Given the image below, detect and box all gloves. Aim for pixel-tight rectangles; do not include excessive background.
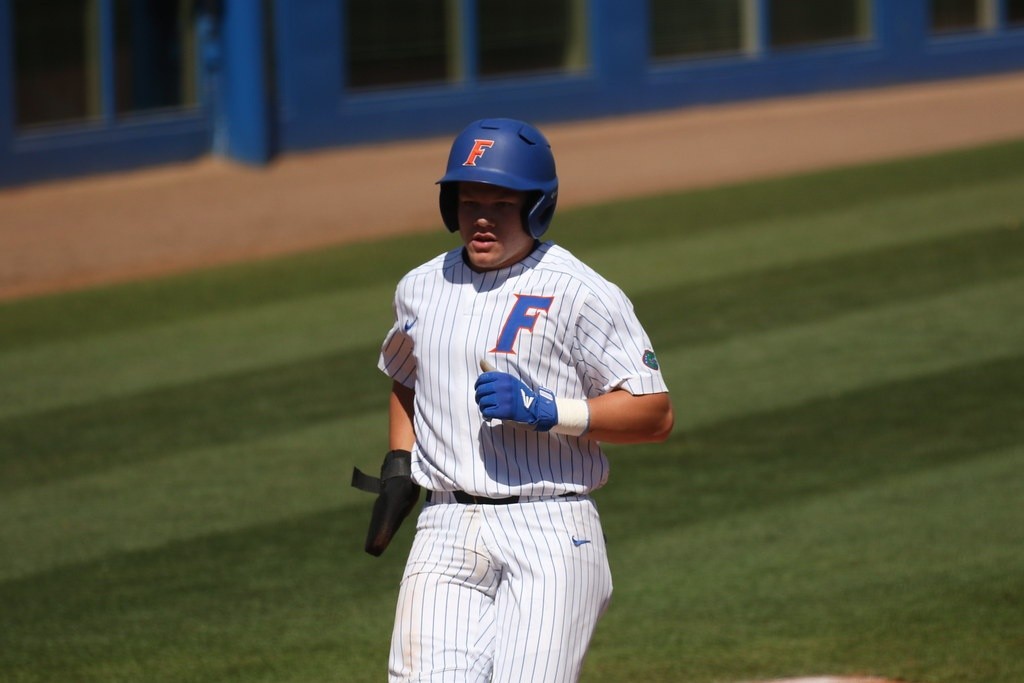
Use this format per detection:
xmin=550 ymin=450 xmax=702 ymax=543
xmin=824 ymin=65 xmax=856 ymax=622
xmin=473 ymin=359 xmax=559 ymax=432
xmin=350 ymin=448 xmax=422 ymax=557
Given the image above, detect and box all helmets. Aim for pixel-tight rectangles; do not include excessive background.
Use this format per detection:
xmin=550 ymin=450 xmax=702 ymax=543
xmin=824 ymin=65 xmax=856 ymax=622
xmin=435 ymin=119 xmax=559 ymax=240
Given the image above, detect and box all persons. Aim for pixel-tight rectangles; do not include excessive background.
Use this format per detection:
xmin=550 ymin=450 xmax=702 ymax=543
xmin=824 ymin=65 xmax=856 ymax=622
xmin=365 ymin=117 xmax=674 ymax=683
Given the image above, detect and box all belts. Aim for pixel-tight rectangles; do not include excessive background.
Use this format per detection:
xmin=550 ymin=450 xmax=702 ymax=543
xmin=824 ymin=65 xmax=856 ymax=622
xmin=426 ymin=490 xmax=578 ymax=506
